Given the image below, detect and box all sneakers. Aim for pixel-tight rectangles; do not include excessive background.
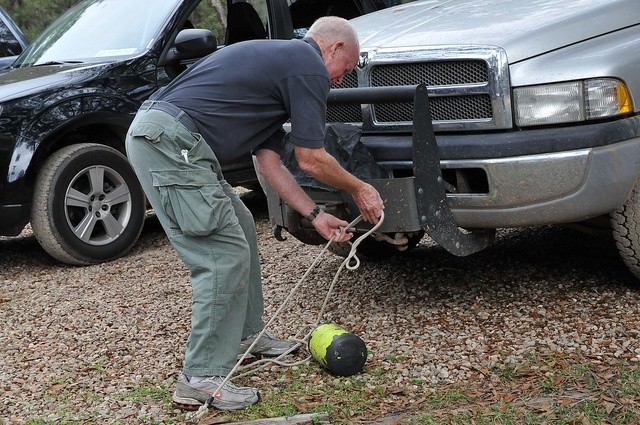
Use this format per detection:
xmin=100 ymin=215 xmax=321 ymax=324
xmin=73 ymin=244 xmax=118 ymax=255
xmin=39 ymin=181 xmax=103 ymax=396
xmin=172 ymin=374 xmax=261 ymax=411
xmin=236 ymin=330 xmax=302 ymax=364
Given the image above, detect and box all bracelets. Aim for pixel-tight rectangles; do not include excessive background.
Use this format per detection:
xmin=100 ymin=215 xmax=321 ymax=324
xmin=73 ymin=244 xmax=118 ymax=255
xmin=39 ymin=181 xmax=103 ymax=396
xmin=305 ymin=206 xmax=320 ymax=222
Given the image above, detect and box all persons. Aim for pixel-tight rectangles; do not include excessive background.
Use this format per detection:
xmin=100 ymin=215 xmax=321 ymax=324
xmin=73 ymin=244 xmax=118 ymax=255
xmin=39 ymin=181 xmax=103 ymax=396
xmin=124 ymin=16 xmax=385 ymax=412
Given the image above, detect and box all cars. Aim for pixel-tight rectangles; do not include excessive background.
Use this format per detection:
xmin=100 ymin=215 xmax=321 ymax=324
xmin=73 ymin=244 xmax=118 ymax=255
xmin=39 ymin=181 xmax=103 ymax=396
xmin=0 ymin=0 xmax=412 ymax=265
xmin=252 ymin=0 xmax=639 ymax=282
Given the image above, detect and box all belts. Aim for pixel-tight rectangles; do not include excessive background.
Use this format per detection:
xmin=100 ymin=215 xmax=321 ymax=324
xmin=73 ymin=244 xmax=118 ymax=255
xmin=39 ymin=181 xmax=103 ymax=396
xmin=139 ymin=101 xmax=199 ymax=133
